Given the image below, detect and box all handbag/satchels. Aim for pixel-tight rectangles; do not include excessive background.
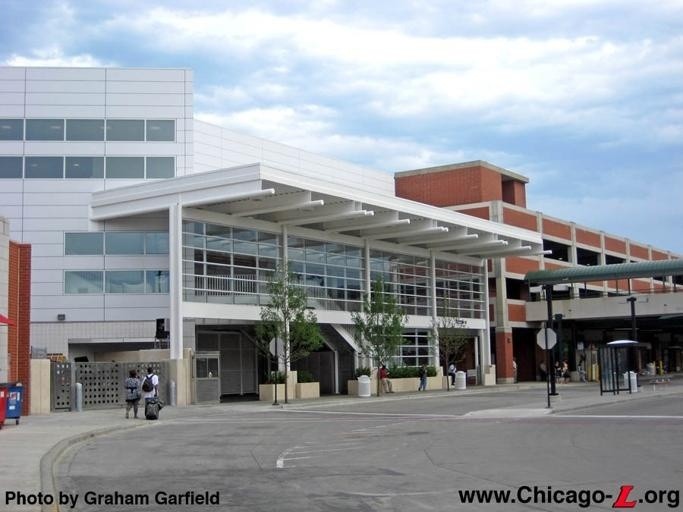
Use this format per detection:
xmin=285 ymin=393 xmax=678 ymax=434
xmin=145 ymin=397 xmax=160 ymax=404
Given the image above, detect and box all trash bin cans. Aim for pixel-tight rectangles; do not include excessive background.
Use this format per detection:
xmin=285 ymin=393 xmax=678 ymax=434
xmin=455 ymin=371 xmax=467 ymax=390
xmin=622 ymin=371 xmax=638 ymax=393
xmin=0 ymin=383 xmax=24 ymax=430
xmin=357 ymin=375 xmax=371 ymax=398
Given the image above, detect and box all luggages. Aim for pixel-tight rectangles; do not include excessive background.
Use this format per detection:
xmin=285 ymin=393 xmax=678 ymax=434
xmin=145 ymin=404 xmax=159 ymax=420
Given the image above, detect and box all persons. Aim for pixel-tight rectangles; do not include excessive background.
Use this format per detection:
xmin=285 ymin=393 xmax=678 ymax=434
xmin=539 ymin=358 xmax=588 ymax=383
xmin=124 ymin=368 xmax=141 ymax=419
xmin=512 ymin=357 xmax=517 ymax=384
xmin=448 ymin=362 xmax=457 ymax=385
xmin=417 ymin=363 xmax=427 ymax=391
xmin=379 ymin=363 xmax=395 ymax=393
xmin=139 ymin=366 xmax=160 ymax=418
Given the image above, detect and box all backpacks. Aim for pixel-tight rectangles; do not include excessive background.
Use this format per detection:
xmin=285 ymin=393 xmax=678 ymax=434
xmin=143 ymin=375 xmax=154 ymax=392
